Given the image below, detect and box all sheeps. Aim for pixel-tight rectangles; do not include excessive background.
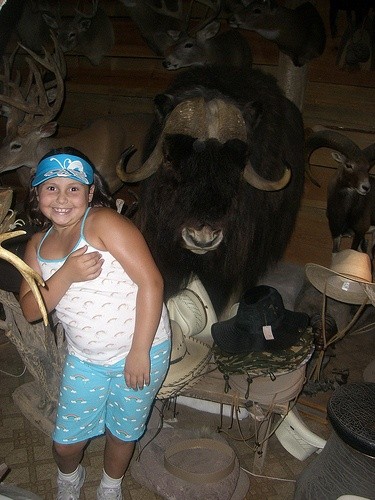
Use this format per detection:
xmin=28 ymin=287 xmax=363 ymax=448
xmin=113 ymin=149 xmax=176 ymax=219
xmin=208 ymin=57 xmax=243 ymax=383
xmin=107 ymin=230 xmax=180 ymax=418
xmin=306 ymin=130 xmax=375 ymax=259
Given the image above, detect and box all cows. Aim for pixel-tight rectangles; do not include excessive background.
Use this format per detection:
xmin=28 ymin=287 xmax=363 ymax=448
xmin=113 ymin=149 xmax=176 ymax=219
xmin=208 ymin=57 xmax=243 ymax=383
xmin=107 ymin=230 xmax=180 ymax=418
xmin=115 ymin=63 xmax=306 ymax=320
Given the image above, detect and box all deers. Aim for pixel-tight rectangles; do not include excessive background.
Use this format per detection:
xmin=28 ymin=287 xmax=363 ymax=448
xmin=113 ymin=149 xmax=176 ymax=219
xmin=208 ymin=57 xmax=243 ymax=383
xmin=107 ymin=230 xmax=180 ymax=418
xmin=145 ymin=1 xmax=253 ymax=70
xmin=226 ymin=0 xmax=325 ymax=67
xmin=30 ymin=0 xmax=114 ymax=79
xmin=0 ymin=42 xmax=155 ymax=196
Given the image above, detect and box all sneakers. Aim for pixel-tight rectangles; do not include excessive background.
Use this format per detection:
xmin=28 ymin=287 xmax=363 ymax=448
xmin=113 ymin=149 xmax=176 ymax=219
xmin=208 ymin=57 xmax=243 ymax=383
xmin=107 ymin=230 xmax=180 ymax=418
xmin=95 ymin=476 xmax=126 ymax=500
xmin=54 ymin=463 xmax=87 ymax=499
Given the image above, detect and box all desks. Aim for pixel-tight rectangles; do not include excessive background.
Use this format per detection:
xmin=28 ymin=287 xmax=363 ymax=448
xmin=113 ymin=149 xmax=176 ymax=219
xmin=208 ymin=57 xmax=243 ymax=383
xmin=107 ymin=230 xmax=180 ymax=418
xmin=185 ymin=365 xmax=306 ymax=476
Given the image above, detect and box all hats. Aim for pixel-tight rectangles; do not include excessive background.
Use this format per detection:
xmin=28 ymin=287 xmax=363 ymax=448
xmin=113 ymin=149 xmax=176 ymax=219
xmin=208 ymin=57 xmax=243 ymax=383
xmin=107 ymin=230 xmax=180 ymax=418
xmin=211 ymin=285 xmax=317 ymax=403
xmin=273 ymin=403 xmax=326 ymax=461
xmin=305 ymin=248 xmax=375 ymax=305
xmin=32 ymin=154 xmax=93 ymax=187
xmin=151 ymin=266 xmax=218 ymax=399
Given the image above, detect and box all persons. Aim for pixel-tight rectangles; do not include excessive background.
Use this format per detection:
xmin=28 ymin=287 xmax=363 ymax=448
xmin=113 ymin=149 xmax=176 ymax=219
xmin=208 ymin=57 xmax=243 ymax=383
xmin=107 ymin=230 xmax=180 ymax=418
xmin=20 ymin=146 xmax=172 ymax=500
